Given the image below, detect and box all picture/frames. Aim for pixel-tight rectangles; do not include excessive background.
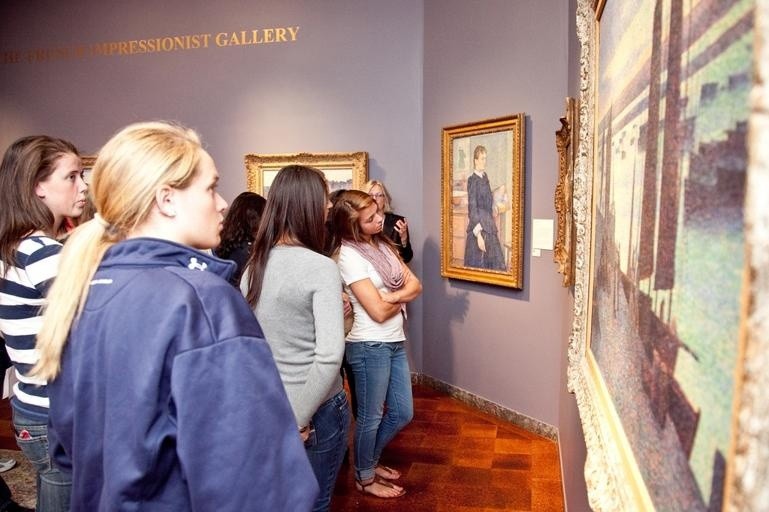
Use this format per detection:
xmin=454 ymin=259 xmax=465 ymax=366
xmin=244 ymin=150 xmax=369 ymax=199
xmin=440 ymin=112 xmax=528 ymax=290
xmin=553 ymin=0 xmax=769 ymax=512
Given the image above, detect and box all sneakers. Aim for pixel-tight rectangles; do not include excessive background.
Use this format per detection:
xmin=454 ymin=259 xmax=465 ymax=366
xmin=0 ymin=458 xmax=17 ymax=473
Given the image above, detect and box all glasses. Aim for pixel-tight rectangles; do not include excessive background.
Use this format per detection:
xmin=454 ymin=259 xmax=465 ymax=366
xmin=369 ymin=194 xmax=383 ymax=198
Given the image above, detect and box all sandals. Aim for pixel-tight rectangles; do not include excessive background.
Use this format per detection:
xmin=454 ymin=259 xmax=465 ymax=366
xmin=356 ymin=463 xmax=406 ymax=498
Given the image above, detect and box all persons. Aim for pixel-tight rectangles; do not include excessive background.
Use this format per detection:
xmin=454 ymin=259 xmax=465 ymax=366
xmin=0 ymin=134 xmax=90 ymax=512
xmin=216 ymin=164 xmax=426 ymax=511
xmin=46 ymin=120 xmax=322 ymax=512
xmin=462 ymin=146 xmax=508 ymax=274
